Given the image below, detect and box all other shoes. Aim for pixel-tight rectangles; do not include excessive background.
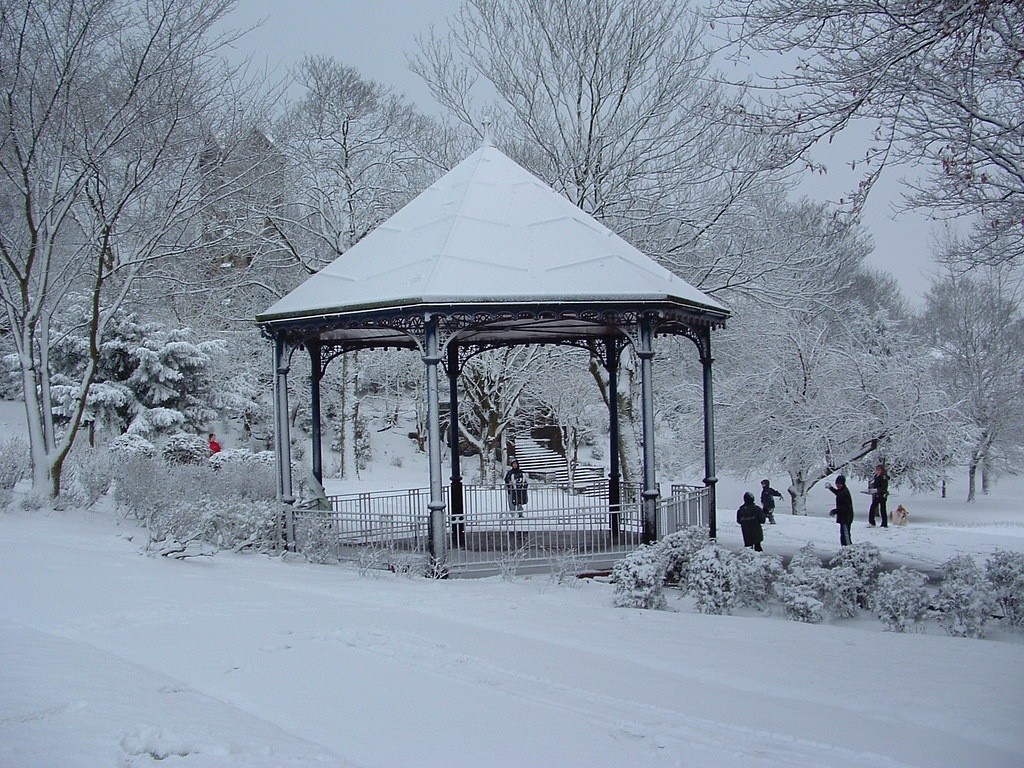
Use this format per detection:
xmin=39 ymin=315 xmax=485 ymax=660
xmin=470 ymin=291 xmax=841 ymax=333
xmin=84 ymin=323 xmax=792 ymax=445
xmin=881 ymin=526 xmax=886 ymax=528
xmin=866 ymin=524 xmax=876 ymax=528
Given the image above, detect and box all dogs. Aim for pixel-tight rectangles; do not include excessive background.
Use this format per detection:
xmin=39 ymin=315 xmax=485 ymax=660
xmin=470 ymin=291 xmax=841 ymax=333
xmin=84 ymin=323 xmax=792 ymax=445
xmin=890 ymin=504 xmax=909 ymax=524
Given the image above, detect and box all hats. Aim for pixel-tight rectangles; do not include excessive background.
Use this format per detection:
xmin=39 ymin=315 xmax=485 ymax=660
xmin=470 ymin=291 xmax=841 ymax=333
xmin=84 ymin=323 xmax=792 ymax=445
xmin=836 ymin=475 xmax=846 ymax=483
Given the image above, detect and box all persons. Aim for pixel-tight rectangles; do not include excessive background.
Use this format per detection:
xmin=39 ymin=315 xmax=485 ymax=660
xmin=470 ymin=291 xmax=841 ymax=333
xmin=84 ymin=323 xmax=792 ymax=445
xmin=209 ymin=433 xmax=222 ymax=457
xmin=760 ymin=479 xmax=783 ymax=524
xmin=505 ymin=458 xmax=528 ymax=518
xmin=860 ymin=465 xmax=888 ymax=529
xmin=824 ymin=475 xmax=854 ymax=547
xmin=736 ymin=492 xmax=766 ymax=553
